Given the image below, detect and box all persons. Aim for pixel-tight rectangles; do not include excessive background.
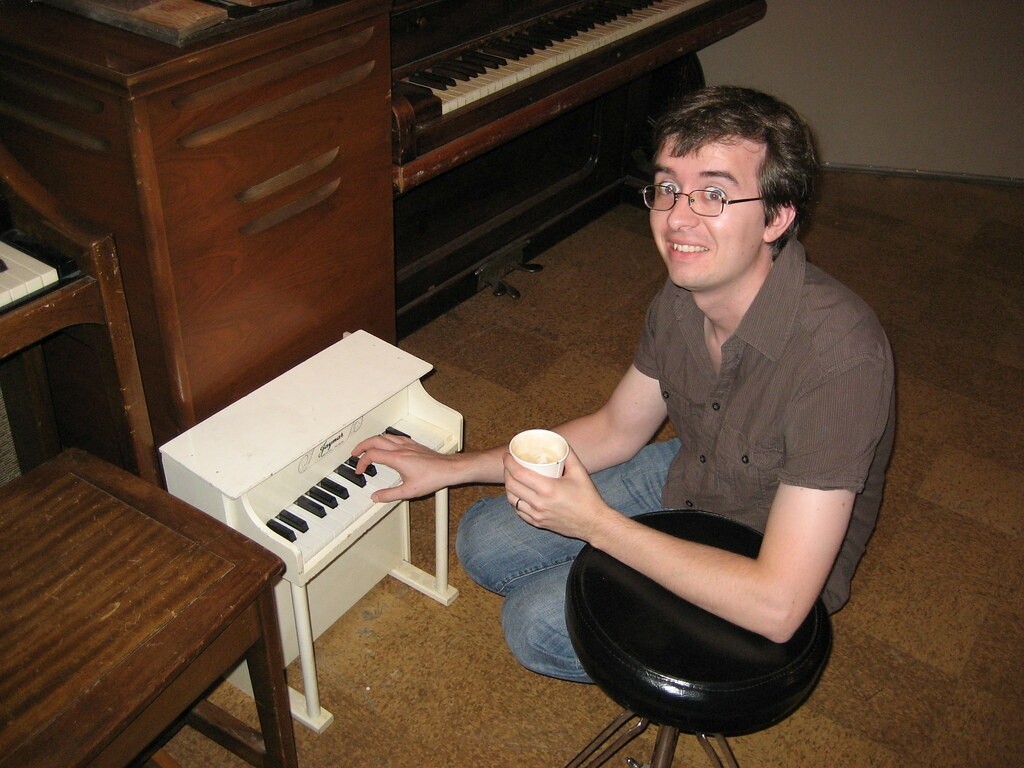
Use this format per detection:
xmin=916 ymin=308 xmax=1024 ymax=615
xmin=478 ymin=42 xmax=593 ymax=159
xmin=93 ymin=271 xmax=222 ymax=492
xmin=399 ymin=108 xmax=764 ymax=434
xmin=352 ymin=86 xmax=897 ymax=682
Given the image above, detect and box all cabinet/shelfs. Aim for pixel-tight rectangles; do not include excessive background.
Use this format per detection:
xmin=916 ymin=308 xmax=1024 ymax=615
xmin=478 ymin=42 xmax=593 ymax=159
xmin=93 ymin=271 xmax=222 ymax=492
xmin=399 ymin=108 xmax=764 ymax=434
xmin=0 ymin=0 xmax=397 ymax=493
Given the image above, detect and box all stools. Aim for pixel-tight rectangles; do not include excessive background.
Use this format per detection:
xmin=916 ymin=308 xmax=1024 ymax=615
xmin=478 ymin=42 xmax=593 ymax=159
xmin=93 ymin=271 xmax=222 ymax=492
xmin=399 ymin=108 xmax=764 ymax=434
xmin=563 ymin=508 xmax=833 ymax=768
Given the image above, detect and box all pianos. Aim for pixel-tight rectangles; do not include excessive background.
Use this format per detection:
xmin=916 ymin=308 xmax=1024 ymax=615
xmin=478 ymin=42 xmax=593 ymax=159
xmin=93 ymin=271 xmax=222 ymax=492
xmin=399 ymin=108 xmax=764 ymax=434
xmin=157 ymin=328 xmax=466 ymax=737
xmin=0 ymin=142 xmax=163 ymax=489
xmin=392 ymin=0 xmax=770 ymax=343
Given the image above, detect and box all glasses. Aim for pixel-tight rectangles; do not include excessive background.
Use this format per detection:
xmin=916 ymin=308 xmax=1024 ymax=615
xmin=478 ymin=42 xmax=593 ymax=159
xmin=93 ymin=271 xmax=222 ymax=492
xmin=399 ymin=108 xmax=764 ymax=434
xmin=641 ymin=185 xmax=767 ymax=217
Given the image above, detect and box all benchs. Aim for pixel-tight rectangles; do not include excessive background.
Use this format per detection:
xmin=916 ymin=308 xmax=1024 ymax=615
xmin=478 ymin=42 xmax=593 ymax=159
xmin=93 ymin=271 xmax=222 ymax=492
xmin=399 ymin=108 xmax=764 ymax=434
xmin=0 ymin=445 xmax=299 ymax=768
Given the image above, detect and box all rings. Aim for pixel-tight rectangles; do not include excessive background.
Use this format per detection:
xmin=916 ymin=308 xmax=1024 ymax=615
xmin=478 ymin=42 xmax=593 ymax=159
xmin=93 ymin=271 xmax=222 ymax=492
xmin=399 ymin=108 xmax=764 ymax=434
xmin=516 ymin=498 xmax=521 ymax=510
